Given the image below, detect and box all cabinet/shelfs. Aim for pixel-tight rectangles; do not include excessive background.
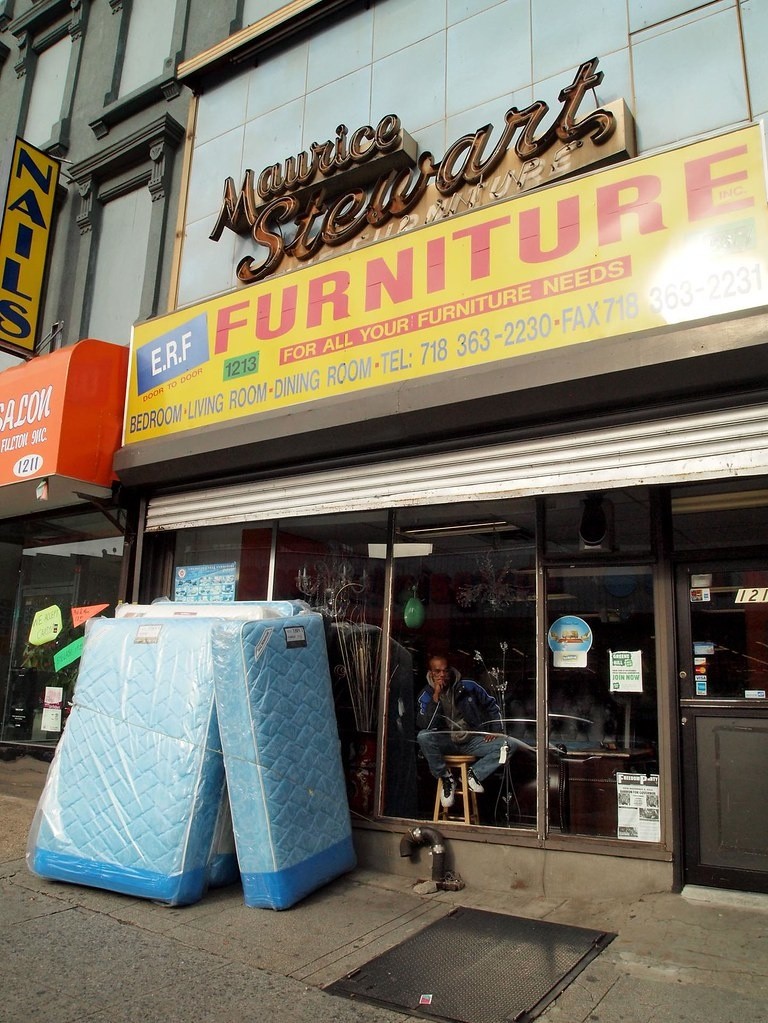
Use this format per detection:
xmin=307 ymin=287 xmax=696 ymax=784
xmin=560 ymin=745 xmax=653 ymax=837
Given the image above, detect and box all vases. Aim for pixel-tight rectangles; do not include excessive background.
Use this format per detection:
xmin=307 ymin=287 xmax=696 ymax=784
xmin=345 ymin=729 xmax=378 ymax=817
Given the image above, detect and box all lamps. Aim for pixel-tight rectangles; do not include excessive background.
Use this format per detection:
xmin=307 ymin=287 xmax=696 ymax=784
xmin=404 ymin=584 xmax=426 ymax=628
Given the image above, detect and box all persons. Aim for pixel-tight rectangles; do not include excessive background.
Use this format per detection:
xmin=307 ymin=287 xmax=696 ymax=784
xmin=619 ymin=794 xmax=658 ymax=836
xmin=415 ymin=654 xmax=517 ymax=807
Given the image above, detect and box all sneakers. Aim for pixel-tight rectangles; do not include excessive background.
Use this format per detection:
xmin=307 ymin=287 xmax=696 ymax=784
xmin=459 ymin=769 xmax=485 ymax=793
xmin=440 ymin=777 xmax=457 ymax=807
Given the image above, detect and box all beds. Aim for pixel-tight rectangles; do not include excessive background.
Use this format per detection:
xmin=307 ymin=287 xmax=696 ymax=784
xmin=27 ymin=600 xmax=358 ymax=913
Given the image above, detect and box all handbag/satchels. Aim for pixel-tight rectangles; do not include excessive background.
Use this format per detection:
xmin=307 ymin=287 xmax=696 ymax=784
xmin=450 ymin=720 xmax=470 ymax=744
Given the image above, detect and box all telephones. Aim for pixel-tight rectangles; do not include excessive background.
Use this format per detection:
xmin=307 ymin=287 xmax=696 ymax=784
xmin=555 ymin=743 xmax=568 ymax=754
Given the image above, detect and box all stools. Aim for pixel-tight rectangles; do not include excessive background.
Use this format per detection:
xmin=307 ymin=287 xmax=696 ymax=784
xmin=433 ymin=754 xmax=480 ymax=825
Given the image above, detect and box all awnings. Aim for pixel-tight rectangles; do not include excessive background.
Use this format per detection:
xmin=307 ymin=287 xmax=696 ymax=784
xmin=0 ymin=339 xmax=130 ymax=519
